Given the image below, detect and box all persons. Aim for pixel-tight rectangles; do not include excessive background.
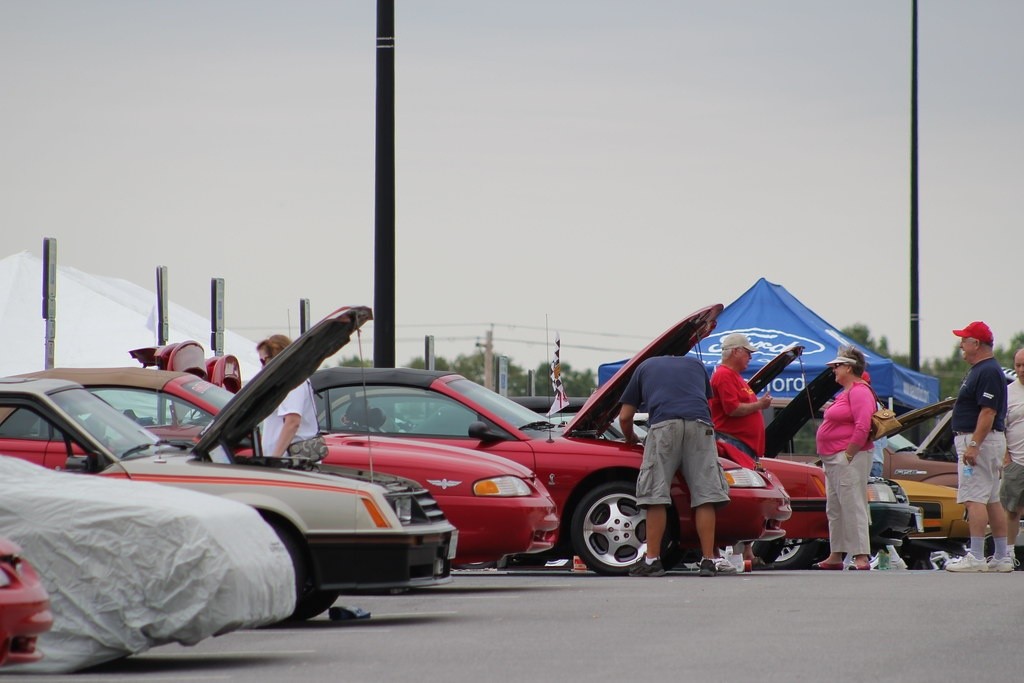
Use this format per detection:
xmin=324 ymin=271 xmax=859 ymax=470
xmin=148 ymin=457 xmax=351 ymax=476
xmin=861 ymin=373 xmax=886 ymax=478
xmin=619 ymin=354 xmax=730 ymax=576
xmin=812 ymin=347 xmax=877 ymax=571
xmin=946 ymin=321 xmax=1013 ymax=573
xmin=257 ymin=335 xmax=321 ymax=463
xmin=707 ymin=334 xmax=772 ymax=570
xmin=1000 ymin=348 xmax=1024 ymax=567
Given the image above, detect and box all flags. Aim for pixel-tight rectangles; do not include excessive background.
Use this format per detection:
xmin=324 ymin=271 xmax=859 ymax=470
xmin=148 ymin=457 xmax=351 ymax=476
xmin=546 ymin=333 xmax=569 ymax=416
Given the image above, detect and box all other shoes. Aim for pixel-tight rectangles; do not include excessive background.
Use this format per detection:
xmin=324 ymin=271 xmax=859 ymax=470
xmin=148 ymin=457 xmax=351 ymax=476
xmin=869 ymin=553 xmax=908 ymax=570
xmin=812 ymin=562 xmax=844 ymax=570
xmin=752 ymin=556 xmax=776 ymax=569
xmin=848 ymin=564 xmax=871 ymax=570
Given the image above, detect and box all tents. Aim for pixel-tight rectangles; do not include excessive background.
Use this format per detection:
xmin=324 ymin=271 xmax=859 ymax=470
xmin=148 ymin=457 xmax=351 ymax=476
xmin=598 ymin=278 xmax=938 ymax=418
xmin=1 ymin=249 xmax=265 ymax=423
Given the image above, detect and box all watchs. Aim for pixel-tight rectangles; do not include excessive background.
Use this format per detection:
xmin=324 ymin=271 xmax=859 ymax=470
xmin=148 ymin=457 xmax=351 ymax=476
xmin=970 ymin=441 xmax=976 ymax=446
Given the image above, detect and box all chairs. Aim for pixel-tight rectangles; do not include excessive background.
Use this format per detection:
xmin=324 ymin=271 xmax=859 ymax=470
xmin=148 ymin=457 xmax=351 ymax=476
xmin=343 ymin=398 xmax=369 ymax=432
xmin=368 ymin=407 xmax=388 ymax=433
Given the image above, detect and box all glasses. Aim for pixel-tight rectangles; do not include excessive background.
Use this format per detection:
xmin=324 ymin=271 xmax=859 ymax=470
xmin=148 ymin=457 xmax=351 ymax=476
xmin=260 ymin=355 xmax=269 ymax=366
xmin=835 ymin=363 xmax=844 ymax=368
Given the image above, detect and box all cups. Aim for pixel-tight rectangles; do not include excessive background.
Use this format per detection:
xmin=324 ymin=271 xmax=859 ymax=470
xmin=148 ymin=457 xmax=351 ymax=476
xmin=573 ymin=555 xmax=588 ymax=571
xmin=744 ymin=560 xmax=753 ymax=572
xmin=725 ymin=553 xmax=743 ymax=572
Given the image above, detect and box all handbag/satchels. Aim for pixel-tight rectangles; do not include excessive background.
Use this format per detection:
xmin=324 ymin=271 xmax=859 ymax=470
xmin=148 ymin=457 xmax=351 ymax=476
xmin=288 ymin=435 xmax=329 ymax=466
xmin=848 ymin=382 xmax=902 ymax=441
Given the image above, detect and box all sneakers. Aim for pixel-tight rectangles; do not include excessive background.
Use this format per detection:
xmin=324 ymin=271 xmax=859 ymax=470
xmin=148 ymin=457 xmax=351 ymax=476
xmin=628 ymin=552 xmax=665 ymax=577
xmin=700 ymin=557 xmax=717 ymax=576
xmin=946 ymin=552 xmax=988 ymax=573
xmin=989 ymin=556 xmax=1020 ymax=572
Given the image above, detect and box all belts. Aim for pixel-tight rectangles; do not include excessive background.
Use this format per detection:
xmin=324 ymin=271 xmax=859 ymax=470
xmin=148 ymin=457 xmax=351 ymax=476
xmin=953 ymin=430 xmax=970 ymax=436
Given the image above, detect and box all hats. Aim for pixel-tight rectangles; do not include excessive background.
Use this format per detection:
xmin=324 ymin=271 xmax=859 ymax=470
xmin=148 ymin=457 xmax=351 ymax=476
xmin=952 ymin=320 xmax=994 ymax=343
xmin=722 ymin=334 xmax=759 ymax=353
xmin=826 ymin=355 xmax=866 ymax=367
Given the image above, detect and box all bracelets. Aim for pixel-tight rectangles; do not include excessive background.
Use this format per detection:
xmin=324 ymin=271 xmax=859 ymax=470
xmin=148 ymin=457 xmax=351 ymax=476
xmin=845 ymin=453 xmax=852 ymax=460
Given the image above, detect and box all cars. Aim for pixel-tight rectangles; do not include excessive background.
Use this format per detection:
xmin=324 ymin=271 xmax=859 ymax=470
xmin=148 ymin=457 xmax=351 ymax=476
xmin=142 ymin=365 xmax=794 ymax=578
xmin=0 ymin=454 xmax=298 ymax=676
xmin=319 ymin=397 xmax=1024 ymax=578
xmin=2 ymin=366 xmax=562 ymax=572
xmin=1 ymin=304 xmax=458 ymax=632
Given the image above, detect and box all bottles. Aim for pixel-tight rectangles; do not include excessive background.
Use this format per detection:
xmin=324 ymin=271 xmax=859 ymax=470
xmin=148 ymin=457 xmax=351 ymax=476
xmin=879 ymin=549 xmax=889 ymax=569
